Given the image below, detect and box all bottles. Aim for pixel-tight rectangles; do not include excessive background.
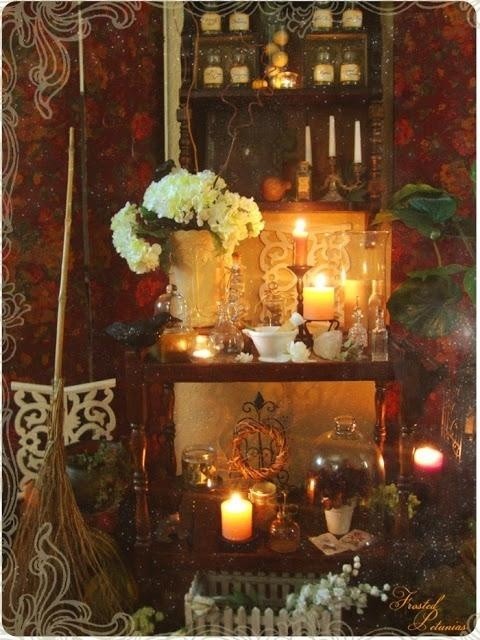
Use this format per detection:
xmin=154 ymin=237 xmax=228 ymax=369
xmin=312 ymin=1 xmax=364 ymax=87
xmin=371 ymin=308 xmax=388 ymax=362
xmin=201 ymin=1 xmax=250 ymax=88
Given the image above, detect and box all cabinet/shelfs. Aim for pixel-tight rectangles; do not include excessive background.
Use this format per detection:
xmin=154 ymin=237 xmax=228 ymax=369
xmin=116 ymin=1 xmax=450 ymax=632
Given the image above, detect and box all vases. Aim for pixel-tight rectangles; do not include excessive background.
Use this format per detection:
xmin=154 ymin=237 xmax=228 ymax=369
xmin=161 ymin=228 xmax=230 ymax=329
xmin=383 ymin=511 xmax=405 ymax=534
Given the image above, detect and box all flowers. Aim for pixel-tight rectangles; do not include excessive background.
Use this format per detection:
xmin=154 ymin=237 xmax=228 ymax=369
xmin=110 ymin=167 xmax=267 ymax=277
xmin=373 ymin=483 xmax=418 ymax=520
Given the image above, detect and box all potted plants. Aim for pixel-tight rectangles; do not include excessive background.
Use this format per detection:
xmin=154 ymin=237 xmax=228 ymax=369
xmin=344 ymin=278 xmax=369 ymax=337
xmin=312 ymin=465 xmax=372 ymax=534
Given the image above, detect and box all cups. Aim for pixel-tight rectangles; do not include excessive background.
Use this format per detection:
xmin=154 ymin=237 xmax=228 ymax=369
xmin=182 ymin=445 xmax=218 ymax=493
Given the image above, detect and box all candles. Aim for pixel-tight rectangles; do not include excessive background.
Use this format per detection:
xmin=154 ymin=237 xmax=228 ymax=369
xmin=304 ymin=124 xmax=313 ymax=168
xmin=302 ymin=273 xmax=335 ymax=322
xmin=221 ymin=490 xmax=253 ymax=542
xmin=293 ymin=217 xmax=309 ymax=268
xmin=328 ymin=115 xmax=337 ymax=157
xmin=354 ymin=120 xmax=363 ymax=164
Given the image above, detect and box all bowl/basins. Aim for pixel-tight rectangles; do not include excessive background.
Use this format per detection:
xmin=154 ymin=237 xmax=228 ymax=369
xmin=242 ymin=326 xmax=298 ymax=362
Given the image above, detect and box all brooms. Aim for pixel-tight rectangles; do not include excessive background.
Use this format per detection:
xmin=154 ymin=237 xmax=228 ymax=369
xmin=1 ymin=127 xmax=131 ymax=636
